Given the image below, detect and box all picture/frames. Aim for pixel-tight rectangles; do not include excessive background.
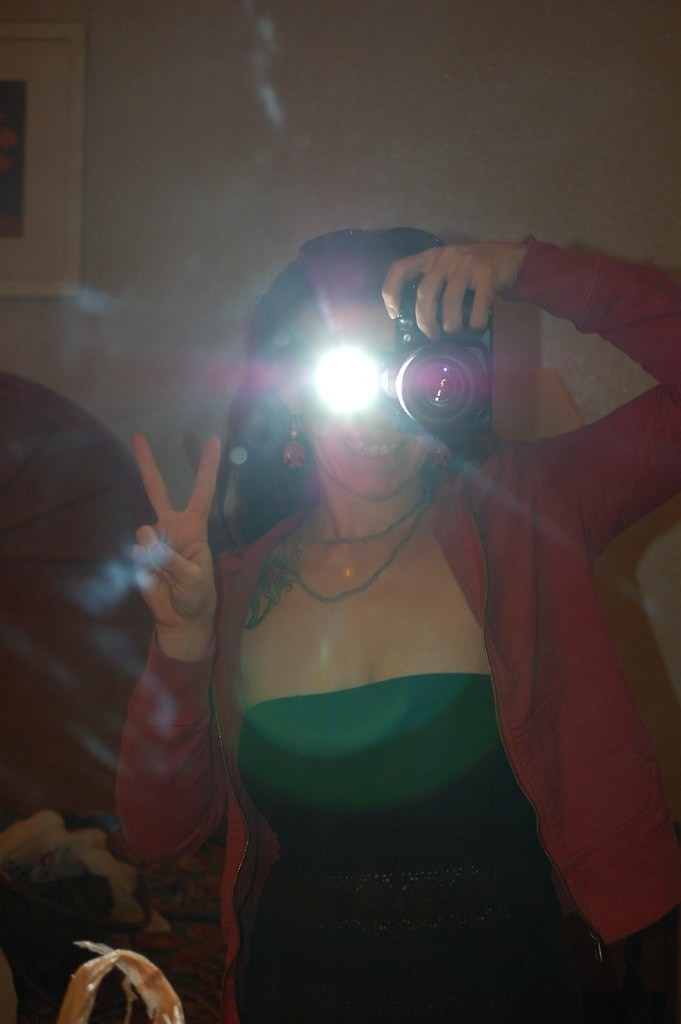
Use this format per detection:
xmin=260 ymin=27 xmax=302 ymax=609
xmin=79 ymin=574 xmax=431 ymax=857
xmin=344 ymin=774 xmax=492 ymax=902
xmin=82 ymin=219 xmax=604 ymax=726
xmin=0 ymin=21 xmax=89 ymax=300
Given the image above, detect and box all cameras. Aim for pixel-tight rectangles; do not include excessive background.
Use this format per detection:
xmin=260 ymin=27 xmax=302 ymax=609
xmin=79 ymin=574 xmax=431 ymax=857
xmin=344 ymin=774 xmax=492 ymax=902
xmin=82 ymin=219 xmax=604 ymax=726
xmin=395 ymin=277 xmax=492 ymax=437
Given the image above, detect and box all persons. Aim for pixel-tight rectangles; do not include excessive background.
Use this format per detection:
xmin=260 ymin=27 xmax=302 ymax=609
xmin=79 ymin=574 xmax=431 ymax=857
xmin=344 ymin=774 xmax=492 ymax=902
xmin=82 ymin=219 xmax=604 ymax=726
xmin=0 ymin=373 xmax=157 ymax=1024
xmin=111 ymin=225 xmax=680 ymax=1024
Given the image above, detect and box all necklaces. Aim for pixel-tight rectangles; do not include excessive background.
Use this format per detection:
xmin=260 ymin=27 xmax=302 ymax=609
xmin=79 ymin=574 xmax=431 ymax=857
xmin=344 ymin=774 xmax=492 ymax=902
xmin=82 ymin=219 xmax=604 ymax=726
xmin=290 ymin=484 xmax=427 ymax=603
